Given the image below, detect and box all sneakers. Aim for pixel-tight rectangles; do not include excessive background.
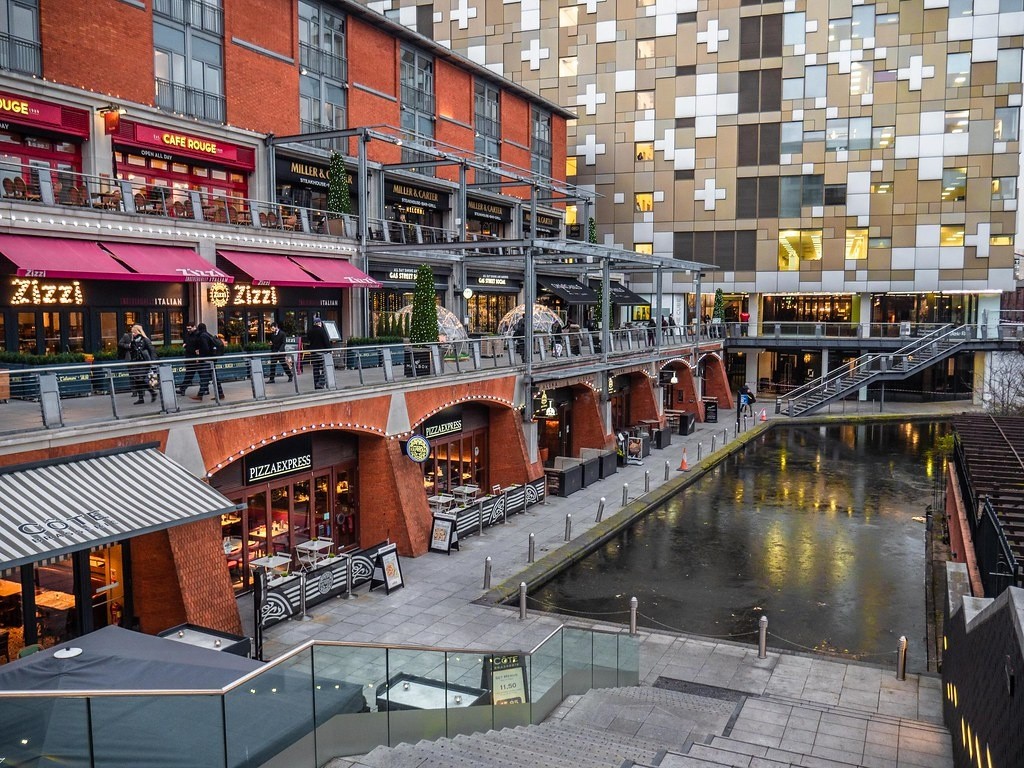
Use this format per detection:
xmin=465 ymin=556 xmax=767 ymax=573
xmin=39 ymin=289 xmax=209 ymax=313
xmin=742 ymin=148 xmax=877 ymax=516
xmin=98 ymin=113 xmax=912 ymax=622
xmin=134 ymin=399 xmax=144 ymax=404
xmin=265 ymin=379 xmax=275 ymax=384
xmin=288 ymin=376 xmax=293 ymax=382
xmin=316 ymin=383 xmax=324 ymax=388
xmin=211 ymin=394 xmax=225 ymax=400
xmin=151 ymin=392 xmax=158 ymax=402
xmin=176 ymin=390 xmax=185 ymax=396
xmin=192 ymin=396 xmax=202 ymax=401
xmin=204 ymin=391 xmax=210 ymax=395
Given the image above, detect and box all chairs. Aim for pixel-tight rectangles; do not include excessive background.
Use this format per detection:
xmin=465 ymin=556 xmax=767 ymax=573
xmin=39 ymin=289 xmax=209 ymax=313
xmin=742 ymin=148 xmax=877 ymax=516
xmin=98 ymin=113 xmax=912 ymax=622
xmin=0 ymin=629 xmax=12 ymax=664
xmin=1 ymin=175 xmax=296 ymax=232
xmin=216 ymin=464 xmax=525 ymax=596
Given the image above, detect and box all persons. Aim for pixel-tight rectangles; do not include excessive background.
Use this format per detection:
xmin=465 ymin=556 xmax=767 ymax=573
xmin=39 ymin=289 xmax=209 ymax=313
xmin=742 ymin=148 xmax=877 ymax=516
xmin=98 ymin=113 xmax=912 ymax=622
xmin=512 ymin=323 xmax=526 ymax=363
xmin=647 ymin=318 xmax=657 ymax=347
xmin=117 ymin=324 xmax=146 ymax=396
xmin=308 ymin=318 xmax=333 ymax=389
xmin=188 ymin=323 xmax=225 ymax=402
xmin=568 ymin=322 xmax=585 ymax=357
xmin=744 ymin=388 xmax=756 ymax=416
xmin=518 ymin=313 xmax=526 ymax=329
xmin=740 ymin=310 xmax=751 ymax=337
xmin=739 ymin=385 xmax=749 ymax=412
xmin=552 ymin=320 xmax=564 ymax=357
xmin=661 ymin=315 xmax=668 ymax=345
xmin=129 ymin=326 xmax=159 ymax=405
xmin=176 ymin=322 xmax=209 ymax=396
xmin=669 ymin=314 xmax=676 ymax=336
xmin=265 ymin=322 xmax=293 ymax=384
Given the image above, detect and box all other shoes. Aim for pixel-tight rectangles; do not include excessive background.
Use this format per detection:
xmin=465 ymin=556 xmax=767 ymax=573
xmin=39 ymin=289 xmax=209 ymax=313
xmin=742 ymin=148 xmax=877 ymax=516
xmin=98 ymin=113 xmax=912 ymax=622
xmin=132 ymin=394 xmax=137 ymax=397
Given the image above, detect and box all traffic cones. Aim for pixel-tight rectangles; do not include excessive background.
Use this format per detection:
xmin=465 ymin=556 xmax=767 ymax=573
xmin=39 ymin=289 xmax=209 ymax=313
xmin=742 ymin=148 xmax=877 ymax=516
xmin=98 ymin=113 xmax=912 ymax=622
xmin=758 ymin=407 xmax=768 ymax=421
xmin=675 ymin=447 xmax=691 ymax=472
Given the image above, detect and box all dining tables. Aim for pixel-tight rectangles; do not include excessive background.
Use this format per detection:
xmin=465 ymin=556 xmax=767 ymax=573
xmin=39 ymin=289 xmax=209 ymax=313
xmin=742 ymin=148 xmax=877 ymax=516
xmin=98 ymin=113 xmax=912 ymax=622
xmin=0 ymin=578 xmax=24 ymax=627
xmin=36 ymin=588 xmax=77 ymax=641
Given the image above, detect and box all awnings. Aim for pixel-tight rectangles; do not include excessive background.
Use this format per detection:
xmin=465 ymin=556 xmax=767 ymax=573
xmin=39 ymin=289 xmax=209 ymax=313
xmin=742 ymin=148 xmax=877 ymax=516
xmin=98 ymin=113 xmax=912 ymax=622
xmin=218 ymin=247 xmax=383 ymax=288
xmin=0 ymin=233 xmax=234 ymax=284
xmin=0 ymin=440 xmax=248 ymax=577
xmin=536 ymin=274 xmax=604 ymax=305
xmin=589 ymin=279 xmax=651 ymax=307
